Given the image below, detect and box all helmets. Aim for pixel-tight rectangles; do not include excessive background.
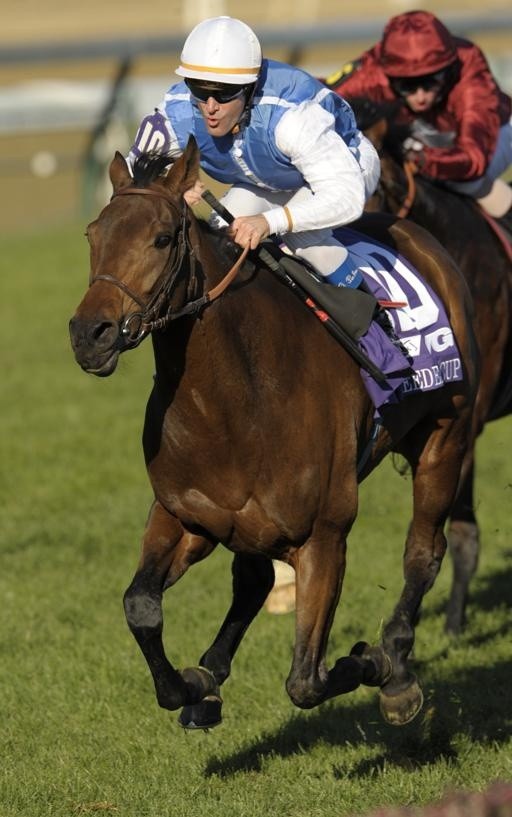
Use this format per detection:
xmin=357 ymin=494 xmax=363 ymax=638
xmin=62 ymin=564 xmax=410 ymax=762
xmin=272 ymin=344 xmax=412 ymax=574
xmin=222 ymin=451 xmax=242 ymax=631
xmin=173 ymin=16 xmax=262 ymax=85
xmin=379 ymin=11 xmax=456 ymax=76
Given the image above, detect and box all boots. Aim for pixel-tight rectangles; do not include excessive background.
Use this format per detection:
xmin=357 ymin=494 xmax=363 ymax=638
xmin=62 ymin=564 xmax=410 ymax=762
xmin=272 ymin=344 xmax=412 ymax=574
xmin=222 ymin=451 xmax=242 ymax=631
xmin=356 ymin=279 xmax=412 ymax=362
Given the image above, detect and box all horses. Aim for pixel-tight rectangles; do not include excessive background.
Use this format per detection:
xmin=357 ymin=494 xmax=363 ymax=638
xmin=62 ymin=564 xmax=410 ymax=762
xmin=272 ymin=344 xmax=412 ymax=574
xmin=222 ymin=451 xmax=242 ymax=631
xmin=68 ymin=91 xmax=512 ymax=730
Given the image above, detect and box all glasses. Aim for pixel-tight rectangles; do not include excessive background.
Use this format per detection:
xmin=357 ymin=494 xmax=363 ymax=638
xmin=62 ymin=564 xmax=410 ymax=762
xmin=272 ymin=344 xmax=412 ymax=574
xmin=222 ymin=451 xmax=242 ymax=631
xmin=184 ymin=78 xmax=245 ymax=103
xmin=395 ymin=72 xmax=443 ymax=95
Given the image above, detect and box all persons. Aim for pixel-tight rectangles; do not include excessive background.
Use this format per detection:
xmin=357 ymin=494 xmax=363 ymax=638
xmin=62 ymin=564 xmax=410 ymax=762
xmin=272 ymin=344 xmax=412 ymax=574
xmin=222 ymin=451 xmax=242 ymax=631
xmin=121 ymin=14 xmax=414 ymax=378
xmin=318 ymin=8 xmax=511 ymax=235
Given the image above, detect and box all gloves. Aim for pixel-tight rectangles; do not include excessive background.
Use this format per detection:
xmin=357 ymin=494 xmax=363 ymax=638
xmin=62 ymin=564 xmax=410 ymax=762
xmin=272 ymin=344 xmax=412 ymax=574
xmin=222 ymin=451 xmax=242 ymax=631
xmin=405 ymin=138 xmax=434 ymax=176
